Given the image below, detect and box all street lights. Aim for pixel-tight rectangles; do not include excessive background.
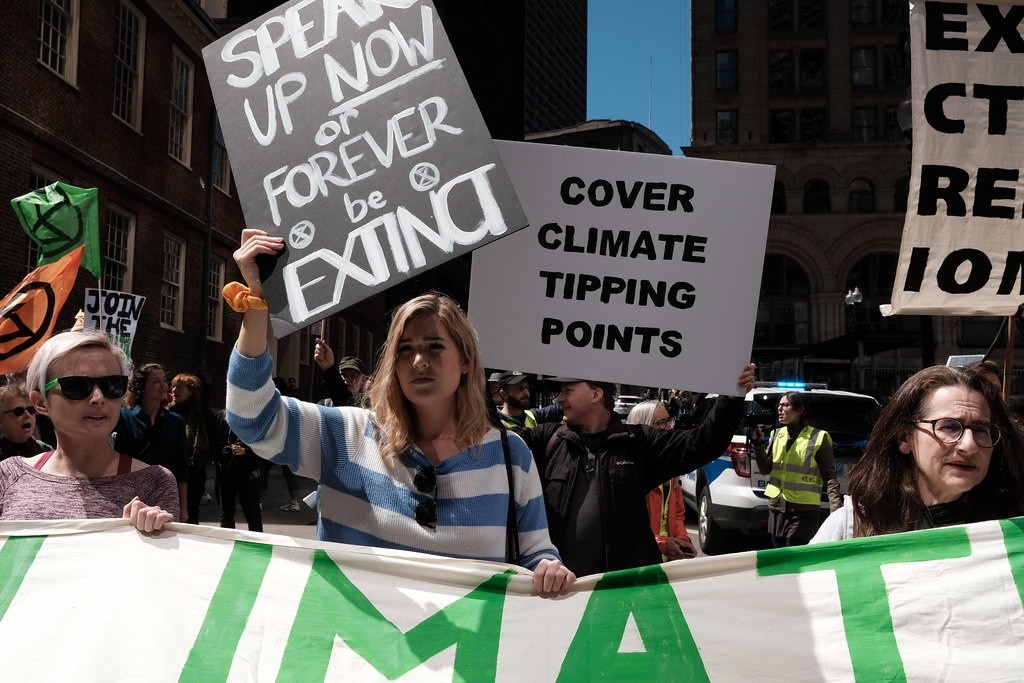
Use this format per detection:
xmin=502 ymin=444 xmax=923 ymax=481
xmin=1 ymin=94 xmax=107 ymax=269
xmin=845 ymin=287 xmax=863 ymax=395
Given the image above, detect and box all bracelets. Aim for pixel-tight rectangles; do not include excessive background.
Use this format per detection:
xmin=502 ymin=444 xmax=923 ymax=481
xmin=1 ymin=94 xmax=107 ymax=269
xmin=221 ymin=281 xmax=268 ymax=312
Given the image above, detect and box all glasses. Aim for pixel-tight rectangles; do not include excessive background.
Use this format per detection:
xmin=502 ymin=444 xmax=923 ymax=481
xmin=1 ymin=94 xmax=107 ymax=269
xmin=914 ymin=418 xmax=1002 ymax=449
xmin=3 ymin=406 xmax=36 ymax=416
xmin=45 ymin=375 xmax=128 ymax=400
xmin=414 ymin=466 xmax=438 ymax=529
xmin=652 ymin=417 xmax=675 ymax=429
xmin=212 ymin=427 xmax=239 ymax=469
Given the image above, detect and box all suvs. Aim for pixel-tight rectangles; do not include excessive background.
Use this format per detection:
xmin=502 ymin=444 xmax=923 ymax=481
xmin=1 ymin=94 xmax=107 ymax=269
xmin=614 ymin=396 xmax=640 ymax=419
xmin=667 ymin=388 xmax=884 ymax=555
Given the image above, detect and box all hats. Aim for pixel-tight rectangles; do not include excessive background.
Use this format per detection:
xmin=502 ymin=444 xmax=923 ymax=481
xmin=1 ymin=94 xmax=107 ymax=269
xmin=497 ymin=371 xmax=528 ymax=395
xmin=487 ymin=373 xmax=501 ymax=381
xmin=339 ymin=356 xmax=365 ymax=374
xmin=544 ymin=377 xmax=617 ymax=396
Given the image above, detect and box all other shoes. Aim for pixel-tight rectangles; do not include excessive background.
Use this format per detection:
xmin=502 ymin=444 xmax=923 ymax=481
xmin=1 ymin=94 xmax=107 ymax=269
xmin=279 ymin=502 xmax=300 ymax=512
xmin=200 ymin=494 xmax=211 ymax=504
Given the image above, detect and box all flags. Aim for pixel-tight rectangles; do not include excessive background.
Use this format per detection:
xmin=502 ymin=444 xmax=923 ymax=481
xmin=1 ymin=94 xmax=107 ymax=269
xmin=11 ymin=180 xmax=101 ymax=278
xmin=0 ymin=243 xmax=85 ymax=374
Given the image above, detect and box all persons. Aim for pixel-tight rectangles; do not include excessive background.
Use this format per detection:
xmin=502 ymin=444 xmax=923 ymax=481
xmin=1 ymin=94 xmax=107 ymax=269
xmin=509 ymin=363 xmax=756 ymax=579
xmin=224 ymin=229 xmax=577 ymax=594
xmin=749 ymin=391 xmax=843 ymax=548
xmin=313 ymin=337 xmax=698 ymax=563
xmin=808 ymin=365 xmax=1024 ymax=544
xmin=0 ymin=330 xmax=180 ymax=531
xmin=0 ymin=364 xmax=300 ymax=532
xmin=963 ymin=360 xmax=1004 ymax=396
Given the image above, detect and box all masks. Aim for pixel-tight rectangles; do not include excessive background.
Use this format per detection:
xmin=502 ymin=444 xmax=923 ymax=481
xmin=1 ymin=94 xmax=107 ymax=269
xmin=498 ymin=388 xmax=527 ymax=410
